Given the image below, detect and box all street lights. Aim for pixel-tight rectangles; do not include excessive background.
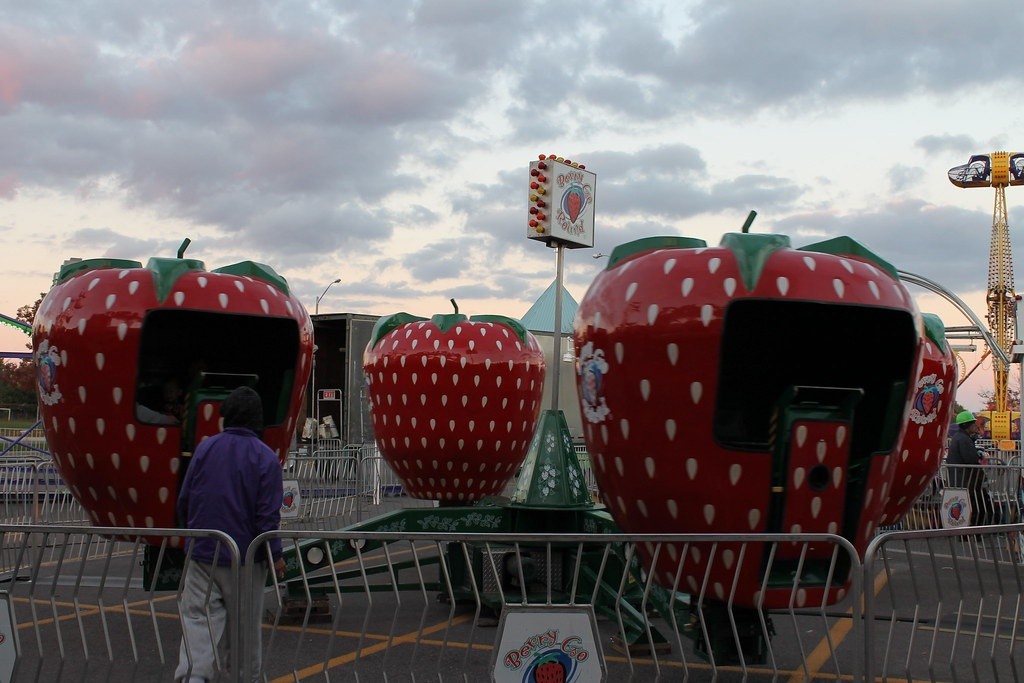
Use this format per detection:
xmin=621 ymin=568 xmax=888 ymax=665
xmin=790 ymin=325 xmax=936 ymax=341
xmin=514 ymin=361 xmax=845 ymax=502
xmin=314 ymin=279 xmax=342 ymax=314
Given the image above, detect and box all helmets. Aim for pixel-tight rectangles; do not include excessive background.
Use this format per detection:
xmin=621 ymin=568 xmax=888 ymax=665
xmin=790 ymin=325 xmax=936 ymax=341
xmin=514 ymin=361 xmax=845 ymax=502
xmin=955 ymin=411 xmax=978 ymax=426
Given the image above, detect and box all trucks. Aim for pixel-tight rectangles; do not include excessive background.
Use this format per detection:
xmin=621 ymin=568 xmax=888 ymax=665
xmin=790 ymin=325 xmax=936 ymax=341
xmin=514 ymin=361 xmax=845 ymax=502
xmin=308 ymin=313 xmax=585 ymax=485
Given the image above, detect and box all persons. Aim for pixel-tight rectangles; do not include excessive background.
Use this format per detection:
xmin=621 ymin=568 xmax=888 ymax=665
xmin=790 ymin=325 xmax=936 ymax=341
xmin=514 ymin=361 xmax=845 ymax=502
xmin=151 ymin=380 xmax=183 ymax=423
xmin=174 ymin=386 xmax=289 ymax=683
xmin=944 ymin=411 xmax=991 ymax=542
